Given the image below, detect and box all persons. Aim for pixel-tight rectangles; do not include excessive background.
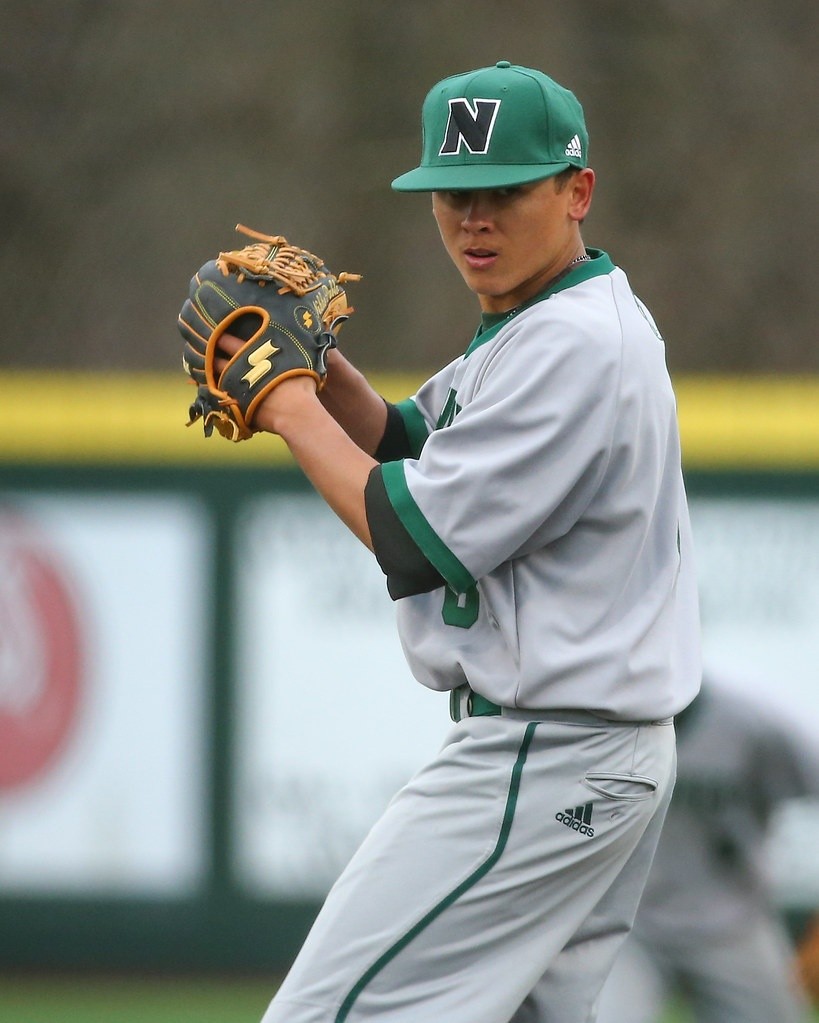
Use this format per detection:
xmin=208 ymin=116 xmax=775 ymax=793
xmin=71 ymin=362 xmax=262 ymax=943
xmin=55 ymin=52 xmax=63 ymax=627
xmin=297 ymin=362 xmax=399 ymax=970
xmin=177 ymin=58 xmax=706 ymax=1023
xmin=615 ymin=678 xmax=819 ymax=1023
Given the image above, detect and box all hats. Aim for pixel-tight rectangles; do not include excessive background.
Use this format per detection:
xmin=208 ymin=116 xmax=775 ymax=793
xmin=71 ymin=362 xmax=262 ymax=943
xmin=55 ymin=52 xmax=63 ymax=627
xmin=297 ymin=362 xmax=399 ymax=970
xmin=391 ymin=60 xmax=589 ymax=193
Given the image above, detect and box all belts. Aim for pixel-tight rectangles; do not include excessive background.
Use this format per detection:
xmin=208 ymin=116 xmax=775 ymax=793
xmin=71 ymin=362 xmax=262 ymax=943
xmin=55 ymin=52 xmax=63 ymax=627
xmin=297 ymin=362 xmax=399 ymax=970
xmin=450 ymin=687 xmax=502 ymax=724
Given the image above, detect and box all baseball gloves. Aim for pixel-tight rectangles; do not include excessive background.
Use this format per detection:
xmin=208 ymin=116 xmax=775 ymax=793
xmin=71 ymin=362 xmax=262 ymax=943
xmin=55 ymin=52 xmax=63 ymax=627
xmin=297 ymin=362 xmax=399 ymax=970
xmin=177 ymin=241 xmax=348 ymax=444
